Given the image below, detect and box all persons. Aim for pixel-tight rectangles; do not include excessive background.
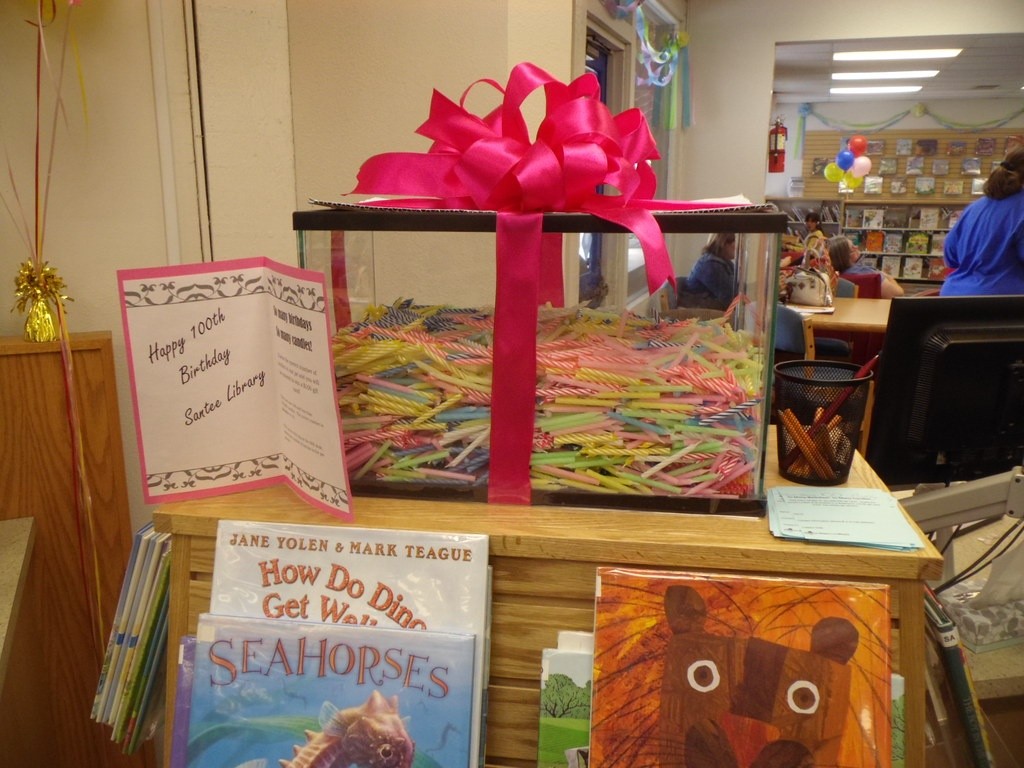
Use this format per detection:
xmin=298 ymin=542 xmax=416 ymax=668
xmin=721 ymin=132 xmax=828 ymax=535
xmin=827 ymin=236 xmax=903 ymax=300
xmin=803 ymin=213 xmax=827 ymax=250
xmin=939 ymin=142 xmax=1024 ymax=296
xmin=685 ymin=233 xmax=746 ymax=311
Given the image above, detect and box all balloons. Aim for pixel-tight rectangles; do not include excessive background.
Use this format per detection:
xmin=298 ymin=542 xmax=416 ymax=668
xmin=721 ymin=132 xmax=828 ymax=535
xmin=825 ymin=136 xmax=871 ymax=190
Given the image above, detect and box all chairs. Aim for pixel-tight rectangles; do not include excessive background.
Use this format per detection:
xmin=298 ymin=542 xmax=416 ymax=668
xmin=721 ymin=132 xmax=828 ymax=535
xmin=841 ymin=274 xmax=883 ymax=296
xmin=837 ymin=278 xmax=859 ymax=298
xmin=776 ymin=304 xmax=877 ymax=461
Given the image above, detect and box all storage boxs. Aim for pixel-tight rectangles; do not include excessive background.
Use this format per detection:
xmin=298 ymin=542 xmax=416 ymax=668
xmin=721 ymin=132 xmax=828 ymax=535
xmin=290 ymin=204 xmax=788 ymax=518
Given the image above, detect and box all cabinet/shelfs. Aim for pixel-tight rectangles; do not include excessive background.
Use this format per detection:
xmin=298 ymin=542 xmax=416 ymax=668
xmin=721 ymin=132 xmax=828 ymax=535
xmin=840 ymin=199 xmax=977 ymax=281
xmin=765 ymin=198 xmax=842 ymax=248
xmin=151 ymin=423 xmax=944 ymax=768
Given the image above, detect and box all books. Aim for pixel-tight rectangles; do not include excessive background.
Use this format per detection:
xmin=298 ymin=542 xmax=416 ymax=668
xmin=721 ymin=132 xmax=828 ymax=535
xmin=838 ymin=135 xmax=1024 ymax=195
xmin=781 ymin=203 xmax=966 ymax=280
xmin=923 ymin=580 xmax=997 ymax=768
xmin=91 ymin=519 xmax=493 ymax=768
xmin=537 ymin=567 xmax=905 ymax=768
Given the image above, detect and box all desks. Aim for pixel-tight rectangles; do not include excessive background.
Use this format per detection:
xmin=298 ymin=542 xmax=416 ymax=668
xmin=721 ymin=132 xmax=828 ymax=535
xmin=785 ymin=297 xmax=892 ymax=344
